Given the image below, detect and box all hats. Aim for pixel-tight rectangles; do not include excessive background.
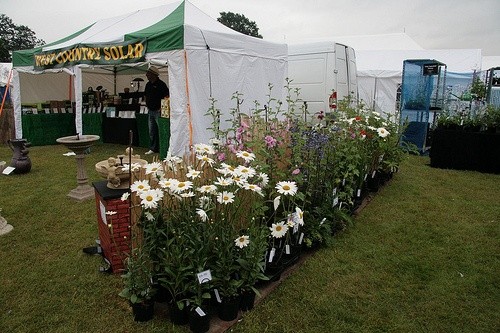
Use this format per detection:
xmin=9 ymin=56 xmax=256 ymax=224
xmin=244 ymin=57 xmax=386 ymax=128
xmin=146 ymin=66 xmax=160 ymax=76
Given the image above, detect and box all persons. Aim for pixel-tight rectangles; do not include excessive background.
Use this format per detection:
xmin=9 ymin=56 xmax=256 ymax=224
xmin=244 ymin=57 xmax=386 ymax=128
xmin=142 ymin=66 xmax=170 ymax=163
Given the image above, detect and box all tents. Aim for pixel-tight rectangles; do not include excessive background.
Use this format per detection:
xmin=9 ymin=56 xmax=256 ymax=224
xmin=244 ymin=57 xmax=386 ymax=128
xmin=357 ymin=33 xmax=500 ymax=134
xmin=0 ymin=1 xmax=289 ymax=165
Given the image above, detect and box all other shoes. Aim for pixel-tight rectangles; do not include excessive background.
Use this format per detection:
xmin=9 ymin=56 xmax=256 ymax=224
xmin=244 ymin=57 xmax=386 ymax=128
xmin=144 ymin=150 xmax=155 ymax=155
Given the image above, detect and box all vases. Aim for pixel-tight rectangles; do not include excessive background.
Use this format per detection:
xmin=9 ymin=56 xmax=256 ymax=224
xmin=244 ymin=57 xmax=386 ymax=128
xmin=216 ymin=294 xmax=241 ymax=321
xmin=266 ymin=260 xmax=280 ymax=282
xmin=367 ymin=178 xmax=381 ymax=192
xmin=238 ymin=287 xmax=255 ymax=310
xmin=168 ymin=298 xmax=211 ymax=333
xmin=129 ymin=296 xmax=155 ymax=322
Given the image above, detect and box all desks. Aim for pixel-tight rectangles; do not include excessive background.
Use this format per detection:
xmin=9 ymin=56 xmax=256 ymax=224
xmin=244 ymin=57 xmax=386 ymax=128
xmin=22 ymin=114 xmax=104 ymax=146
xmin=431 ymin=130 xmax=500 ymax=174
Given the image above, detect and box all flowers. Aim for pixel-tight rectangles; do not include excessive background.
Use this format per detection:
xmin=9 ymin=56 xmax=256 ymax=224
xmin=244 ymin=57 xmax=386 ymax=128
xmin=104 ymin=77 xmax=420 ymax=311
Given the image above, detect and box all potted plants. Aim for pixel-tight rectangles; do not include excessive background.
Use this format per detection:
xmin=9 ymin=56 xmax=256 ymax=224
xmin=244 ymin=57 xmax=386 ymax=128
xmin=438 ymin=104 xmax=500 ymax=134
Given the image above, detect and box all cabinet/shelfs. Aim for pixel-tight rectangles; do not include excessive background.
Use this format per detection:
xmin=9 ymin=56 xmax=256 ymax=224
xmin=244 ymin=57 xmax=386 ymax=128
xmin=398 ymin=59 xmax=447 ymax=155
